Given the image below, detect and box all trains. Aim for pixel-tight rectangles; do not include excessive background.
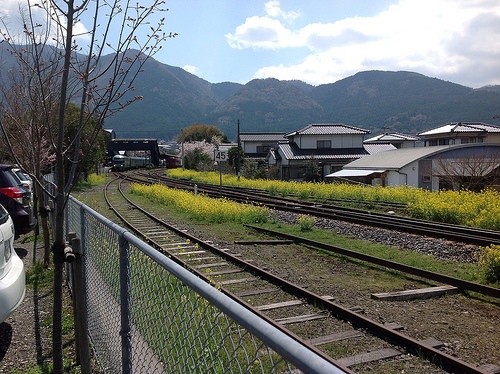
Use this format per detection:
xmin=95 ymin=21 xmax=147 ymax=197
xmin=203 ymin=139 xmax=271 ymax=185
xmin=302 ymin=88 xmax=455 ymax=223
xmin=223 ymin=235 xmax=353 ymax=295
xmin=112 ymin=155 xmax=163 ymax=171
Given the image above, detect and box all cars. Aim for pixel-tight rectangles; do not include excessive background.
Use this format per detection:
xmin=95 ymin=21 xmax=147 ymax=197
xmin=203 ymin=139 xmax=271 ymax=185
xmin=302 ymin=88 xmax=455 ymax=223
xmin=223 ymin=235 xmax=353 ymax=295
xmin=0 ymin=203 xmax=27 ymax=324
xmin=1 ymin=165 xmax=36 ymax=241
xmin=12 ymin=169 xmax=33 ymax=201
